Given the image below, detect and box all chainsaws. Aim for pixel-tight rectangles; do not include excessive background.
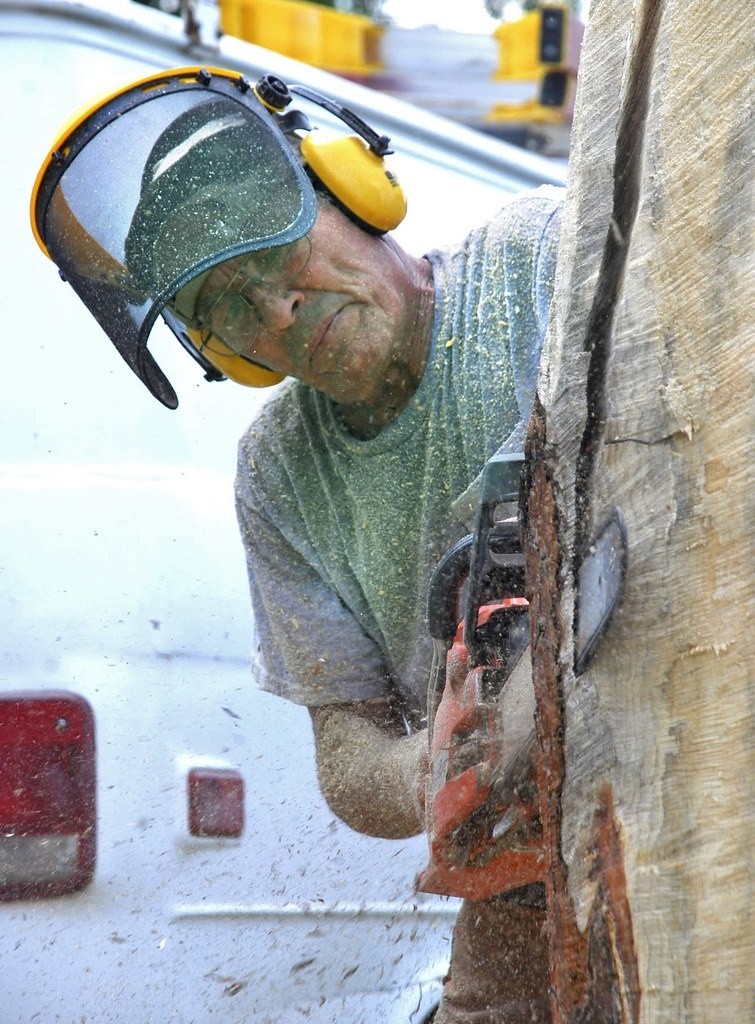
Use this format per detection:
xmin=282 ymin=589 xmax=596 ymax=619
xmin=412 ymin=451 xmax=629 ymax=902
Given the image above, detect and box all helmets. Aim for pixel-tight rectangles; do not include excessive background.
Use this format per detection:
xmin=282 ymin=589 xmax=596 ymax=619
xmin=124 ymin=77 xmax=407 ymax=388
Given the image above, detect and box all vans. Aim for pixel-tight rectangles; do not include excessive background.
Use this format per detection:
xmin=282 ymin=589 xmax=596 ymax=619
xmin=1 ymin=0 xmax=572 ymax=1024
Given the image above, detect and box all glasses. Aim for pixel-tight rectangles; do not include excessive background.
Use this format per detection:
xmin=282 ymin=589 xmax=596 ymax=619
xmin=198 ymin=179 xmax=313 ymax=359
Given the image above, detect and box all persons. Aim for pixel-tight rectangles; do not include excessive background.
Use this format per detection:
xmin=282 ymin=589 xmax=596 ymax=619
xmin=31 ymin=66 xmax=563 ymax=1024
